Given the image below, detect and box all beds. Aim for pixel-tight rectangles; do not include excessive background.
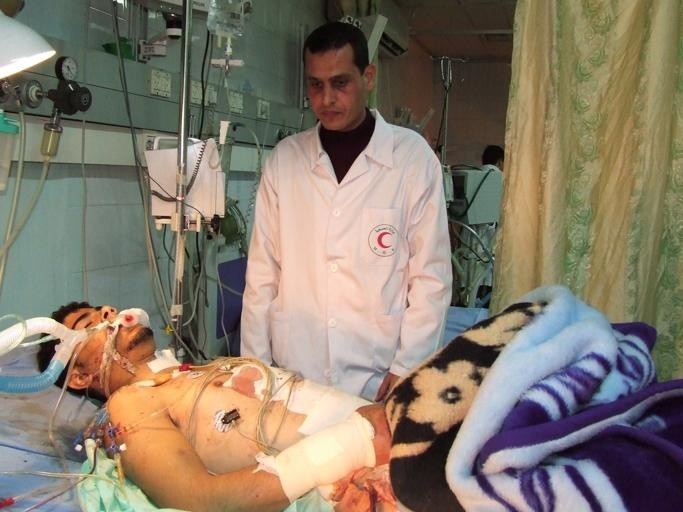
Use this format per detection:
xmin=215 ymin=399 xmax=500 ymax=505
xmin=2 ymin=335 xmax=156 ymax=512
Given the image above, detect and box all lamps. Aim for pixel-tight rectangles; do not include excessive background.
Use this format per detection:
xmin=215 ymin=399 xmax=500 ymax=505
xmin=0 ymin=9 xmax=57 ymax=83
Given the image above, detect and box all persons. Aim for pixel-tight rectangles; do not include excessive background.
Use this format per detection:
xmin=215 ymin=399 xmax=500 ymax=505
xmin=36 ymin=297 xmax=432 ymax=511
xmin=236 ymin=20 xmax=453 ymax=407
xmin=464 ymin=144 xmax=504 ymax=226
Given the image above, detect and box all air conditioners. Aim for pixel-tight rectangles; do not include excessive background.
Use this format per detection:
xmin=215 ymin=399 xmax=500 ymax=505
xmin=358 ymin=1 xmax=409 ymax=59
